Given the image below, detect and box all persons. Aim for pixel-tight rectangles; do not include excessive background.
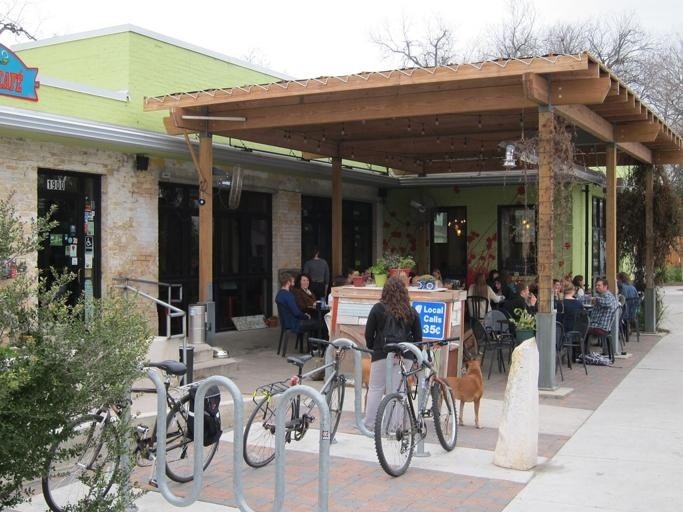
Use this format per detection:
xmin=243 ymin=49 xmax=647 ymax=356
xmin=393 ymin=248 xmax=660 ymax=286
xmin=589 ymin=279 xmax=616 ymax=336
xmin=488 ymin=269 xmax=498 ymax=288
xmin=552 ymin=278 xmax=560 ymax=301
xmin=275 ymin=272 xmax=323 ymax=340
xmin=347 ymin=270 xmax=359 ymax=284
xmin=494 ymin=279 xmax=505 ymax=295
xmin=502 ymin=281 xmax=536 ymax=318
xmin=572 ymin=274 xmax=584 ymax=297
xmin=467 ymin=272 xmax=505 ymax=318
xmin=293 ymin=273 xmax=318 ymax=311
xmin=304 ymin=246 xmax=330 ymax=300
xmin=618 ymin=272 xmax=640 ymax=316
xmin=432 ymin=268 xmax=442 ymax=281
xmin=554 ymin=279 xmax=584 ymax=331
xmin=364 ymin=276 xmax=423 ymax=436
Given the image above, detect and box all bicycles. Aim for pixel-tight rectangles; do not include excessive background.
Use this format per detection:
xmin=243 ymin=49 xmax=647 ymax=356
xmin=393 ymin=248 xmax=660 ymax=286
xmin=374 ymin=337 xmax=460 ymax=476
xmin=243 ymin=337 xmax=374 ymax=468
xmin=41 ymin=359 xmax=221 ymax=512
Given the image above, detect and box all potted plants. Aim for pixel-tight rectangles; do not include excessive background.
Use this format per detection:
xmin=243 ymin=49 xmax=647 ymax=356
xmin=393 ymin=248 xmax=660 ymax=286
xmin=372 ymin=263 xmax=387 ymax=288
xmin=387 ymin=254 xmax=415 ymax=288
xmin=354 ymin=272 xmax=364 ymax=287
xmin=508 ymin=308 xmax=538 ymax=345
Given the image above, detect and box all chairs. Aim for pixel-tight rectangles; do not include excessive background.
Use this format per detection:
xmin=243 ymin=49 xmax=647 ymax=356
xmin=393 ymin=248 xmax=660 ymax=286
xmin=481 ymin=309 xmax=513 ymax=372
xmin=564 ymin=311 xmax=591 ymax=377
xmin=468 ymin=318 xmax=510 ymax=378
xmin=631 ymin=291 xmax=647 ymax=347
xmin=275 ymin=303 xmax=326 ymax=360
xmin=586 ymin=303 xmax=623 ymax=363
xmin=463 ymin=293 xmax=488 ymax=321
xmin=552 ymin=323 xmax=573 ymax=383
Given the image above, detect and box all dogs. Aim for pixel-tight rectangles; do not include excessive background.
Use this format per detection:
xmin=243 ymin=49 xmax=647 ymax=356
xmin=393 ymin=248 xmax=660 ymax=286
xmin=441 ymin=346 xmax=484 ymax=435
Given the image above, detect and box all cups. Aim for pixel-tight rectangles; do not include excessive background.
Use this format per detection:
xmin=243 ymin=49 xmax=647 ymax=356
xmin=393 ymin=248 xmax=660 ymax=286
xmin=321 ymin=297 xmax=325 ymax=308
xmin=528 ymin=293 xmax=534 ymax=299
xmin=586 ymin=294 xmax=592 ymax=306
xmin=576 ymin=295 xmax=581 ymax=303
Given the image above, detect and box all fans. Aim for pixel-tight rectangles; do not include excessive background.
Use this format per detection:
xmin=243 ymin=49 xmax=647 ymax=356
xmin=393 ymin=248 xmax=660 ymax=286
xmin=212 ymin=165 xmax=247 ymax=213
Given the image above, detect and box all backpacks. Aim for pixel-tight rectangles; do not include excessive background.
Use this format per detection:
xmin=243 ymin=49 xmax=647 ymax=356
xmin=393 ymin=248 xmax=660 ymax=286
xmin=380 ymin=300 xmax=408 ymax=354
